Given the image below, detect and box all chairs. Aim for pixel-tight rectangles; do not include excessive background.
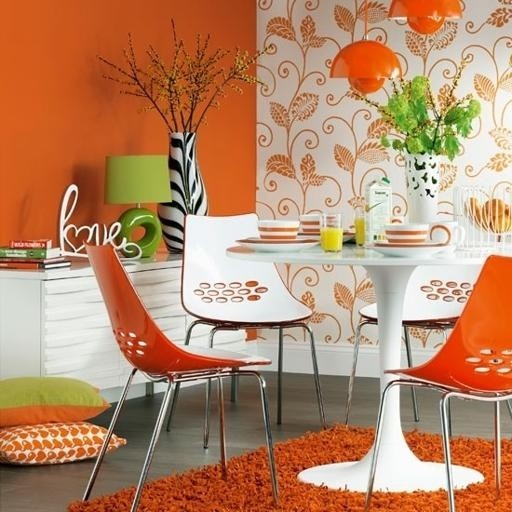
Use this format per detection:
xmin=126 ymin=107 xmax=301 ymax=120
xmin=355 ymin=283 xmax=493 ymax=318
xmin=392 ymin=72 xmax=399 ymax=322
xmin=347 ymin=254 xmax=512 ymax=512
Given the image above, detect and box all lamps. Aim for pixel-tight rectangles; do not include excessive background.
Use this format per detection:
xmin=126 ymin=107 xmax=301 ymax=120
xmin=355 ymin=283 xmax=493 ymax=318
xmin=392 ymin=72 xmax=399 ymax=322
xmin=105 ymin=154 xmax=172 ymax=257
xmin=329 ymin=0 xmax=463 ymax=94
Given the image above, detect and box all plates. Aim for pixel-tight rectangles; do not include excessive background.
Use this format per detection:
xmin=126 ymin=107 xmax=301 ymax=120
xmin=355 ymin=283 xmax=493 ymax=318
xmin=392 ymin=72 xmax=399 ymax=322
xmin=294 ymin=231 xmax=356 ymax=244
xmin=373 ymin=244 xmax=447 ymax=256
xmin=235 ymin=239 xmax=319 ymax=253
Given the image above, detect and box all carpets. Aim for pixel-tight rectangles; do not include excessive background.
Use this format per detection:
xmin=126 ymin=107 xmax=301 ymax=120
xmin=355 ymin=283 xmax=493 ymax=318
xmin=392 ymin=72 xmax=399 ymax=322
xmin=67 ymin=421 xmax=512 ymax=512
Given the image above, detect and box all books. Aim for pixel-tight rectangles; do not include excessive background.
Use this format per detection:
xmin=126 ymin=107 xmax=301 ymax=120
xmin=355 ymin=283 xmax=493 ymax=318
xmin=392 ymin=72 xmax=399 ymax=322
xmin=0 ymin=239 xmax=72 ymax=269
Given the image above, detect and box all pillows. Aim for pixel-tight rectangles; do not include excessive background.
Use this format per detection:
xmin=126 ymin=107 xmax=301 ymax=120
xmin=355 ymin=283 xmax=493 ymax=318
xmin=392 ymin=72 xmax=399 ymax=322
xmin=0 ymin=377 xmax=127 ymax=466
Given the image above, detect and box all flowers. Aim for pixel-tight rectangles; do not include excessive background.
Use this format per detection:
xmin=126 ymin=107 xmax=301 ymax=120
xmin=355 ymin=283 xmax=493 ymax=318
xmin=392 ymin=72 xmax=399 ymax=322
xmin=96 ymin=18 xmax=273 ymax=131
xmin=346 ymin=58 xmax=481 ymax=162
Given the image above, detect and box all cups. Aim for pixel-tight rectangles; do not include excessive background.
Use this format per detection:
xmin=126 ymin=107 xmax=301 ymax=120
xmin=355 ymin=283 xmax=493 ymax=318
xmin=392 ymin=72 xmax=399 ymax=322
xmin=355 ymin=206 xmax=366 ymax=248
xmin=428 ymin=218 xmax=466 ymax=253
xmin=320 ymin=213 xmax=343 ymax=253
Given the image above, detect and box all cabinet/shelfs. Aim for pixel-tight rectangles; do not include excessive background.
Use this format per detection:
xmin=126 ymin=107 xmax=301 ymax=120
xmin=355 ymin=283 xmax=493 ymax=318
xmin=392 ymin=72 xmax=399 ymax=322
xmin=1 ymin=250 xmax=246 ymax=403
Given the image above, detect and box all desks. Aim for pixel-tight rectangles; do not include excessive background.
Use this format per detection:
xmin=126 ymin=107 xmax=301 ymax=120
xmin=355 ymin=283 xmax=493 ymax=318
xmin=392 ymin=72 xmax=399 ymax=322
xmin=227 ymin=247 xmax=512 ymax=496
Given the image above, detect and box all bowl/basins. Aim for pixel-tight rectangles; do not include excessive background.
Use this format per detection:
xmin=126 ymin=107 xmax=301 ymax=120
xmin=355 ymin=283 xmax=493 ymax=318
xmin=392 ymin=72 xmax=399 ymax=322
xmin=256 ymin=219 xmax=300 ymax=239
xmin=384 ymin=224 xmax=429 ymax=243
xmin=300 ymin=214 xmax=326 ymax=232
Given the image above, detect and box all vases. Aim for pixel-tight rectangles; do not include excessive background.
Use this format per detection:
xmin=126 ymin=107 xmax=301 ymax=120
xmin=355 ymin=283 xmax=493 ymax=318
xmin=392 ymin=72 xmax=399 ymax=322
xmin=158 ymin=131 xmax=208 ymax=253
xmin=406 ymin=153 xmax=439 ymax=225
